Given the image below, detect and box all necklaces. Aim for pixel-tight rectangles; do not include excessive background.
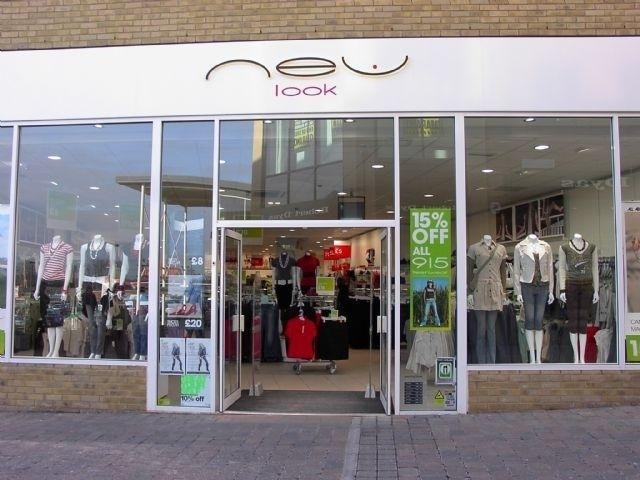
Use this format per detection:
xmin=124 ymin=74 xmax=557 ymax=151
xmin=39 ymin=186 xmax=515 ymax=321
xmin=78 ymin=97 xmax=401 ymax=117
xmin=48 ymin=241 xmax=63 ymax=258
xmin=89 ymin=240 xmax=103 ymax=260
xmin=569 ymin=238 xmax=586 ymax=251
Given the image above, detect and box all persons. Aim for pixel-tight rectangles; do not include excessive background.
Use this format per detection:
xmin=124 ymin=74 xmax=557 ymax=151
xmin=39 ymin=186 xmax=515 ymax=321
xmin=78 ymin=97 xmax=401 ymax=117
xmin=76 ymin=234 xmax=117 ymax=358
xmin=271 ymin=251 xmax=297 ymax=307
xmin=420 ymin=279 xmax=440 ymax=327
xmin=33 ymin=235 xmax=74 ymax=357
xmin=296 ymin=250 xmax=320 ymax=295
xmin=558 ymin=233 xmax=601 ymax=363
xmin=117 ymin=233 xmax=149 ymax=360
xmin=467 ymin=234 xmax=509 ymax=364
xmin=513 ymin=233 xmax=556 ymax=363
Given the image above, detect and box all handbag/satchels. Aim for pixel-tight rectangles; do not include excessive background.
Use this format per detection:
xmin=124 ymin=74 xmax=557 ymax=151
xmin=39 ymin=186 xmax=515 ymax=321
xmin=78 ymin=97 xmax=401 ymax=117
xmin=470 ymin=272 xmax=478 ymax=290
xmin=516 ymin=303 xmax=530 ymax=363
xmin=584 ymin=323 xmax=616 ymax=363
